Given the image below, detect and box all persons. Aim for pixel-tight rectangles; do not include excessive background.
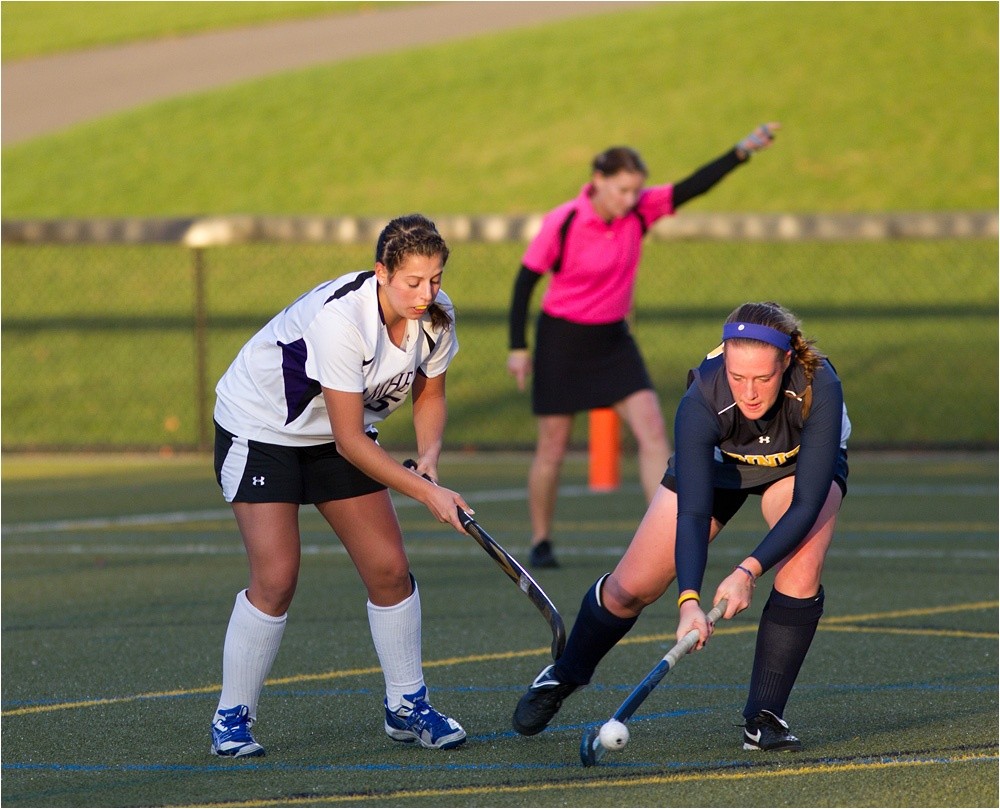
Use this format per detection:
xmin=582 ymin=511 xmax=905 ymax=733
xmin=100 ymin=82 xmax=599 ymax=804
xmin=213 ymin=212 xmax=474 ymax=759
xmin=508 ymin=122 xmax=781 ymax=572
xmin=513 ymin=302 xmax=852 ymax=751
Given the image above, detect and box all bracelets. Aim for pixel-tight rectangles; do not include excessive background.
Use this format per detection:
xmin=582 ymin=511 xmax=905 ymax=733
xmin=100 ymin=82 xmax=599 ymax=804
xmin=736 ymin=566 xmax=756 ymax=583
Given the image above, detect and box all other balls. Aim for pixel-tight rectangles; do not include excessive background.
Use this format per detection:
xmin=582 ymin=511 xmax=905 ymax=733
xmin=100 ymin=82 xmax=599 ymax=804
xmin=599 ymin=720 xmax=629 ymax=750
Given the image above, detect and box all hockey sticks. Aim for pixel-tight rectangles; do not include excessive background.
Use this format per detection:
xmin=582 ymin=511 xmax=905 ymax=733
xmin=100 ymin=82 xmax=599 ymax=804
xmin=401 ymin=457 xmax=568 ymax=662
xmin=580 ymin=602 xmax=731 ymax=762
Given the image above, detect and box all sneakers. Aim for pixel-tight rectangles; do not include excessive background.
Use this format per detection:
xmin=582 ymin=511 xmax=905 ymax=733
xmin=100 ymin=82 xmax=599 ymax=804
xmin=209 ymin=704 xmax=266 ymax=760
xmin=735 ymin=710 xmax=803 ymax=752
xmin=511 ymin=666 xmax=590 ymax=736
xmin=383 ymin=685 xmax=467 ymax=750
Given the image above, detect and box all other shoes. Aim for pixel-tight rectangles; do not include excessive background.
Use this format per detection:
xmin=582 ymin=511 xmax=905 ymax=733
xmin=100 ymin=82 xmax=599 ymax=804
xmin=530 ymin=539 xmax=559 ymax=569
